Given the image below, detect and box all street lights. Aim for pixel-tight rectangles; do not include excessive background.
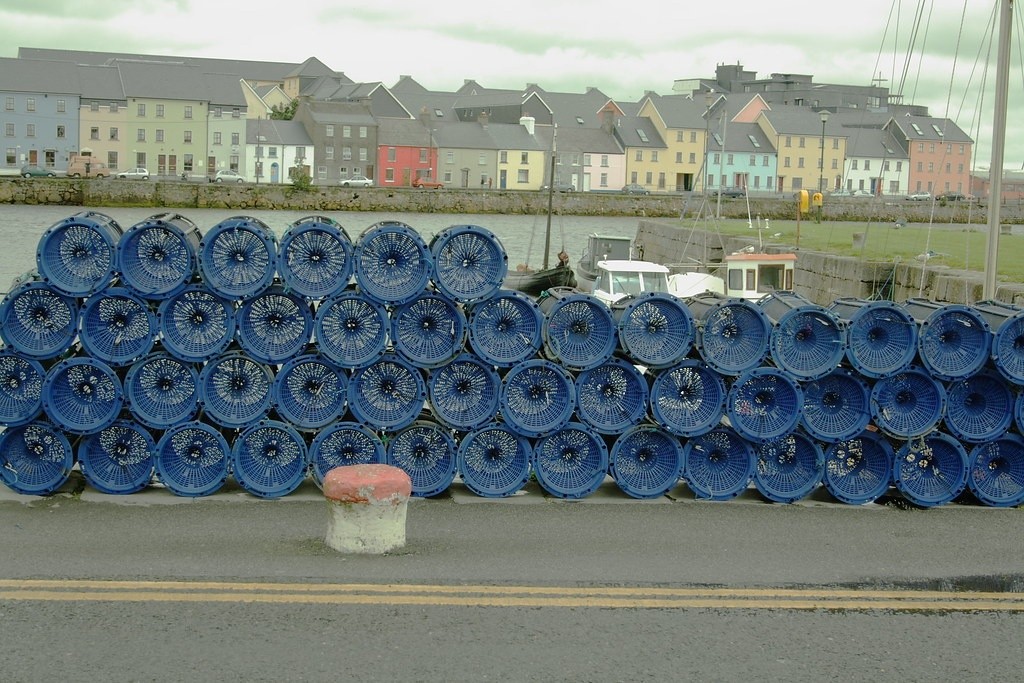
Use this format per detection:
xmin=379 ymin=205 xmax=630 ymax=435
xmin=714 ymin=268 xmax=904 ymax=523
xmin=818 ymin=109 xmax=832 ymax=227
xmin=429 ymin=109 xmax=443 ymax=183
xmin=705 ymin=88 xmax=715 ymax=230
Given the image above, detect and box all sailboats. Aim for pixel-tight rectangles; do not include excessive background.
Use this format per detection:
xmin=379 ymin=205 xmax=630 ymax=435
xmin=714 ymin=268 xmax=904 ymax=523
xmin=502 ymin=122 xmax=578 ymax=297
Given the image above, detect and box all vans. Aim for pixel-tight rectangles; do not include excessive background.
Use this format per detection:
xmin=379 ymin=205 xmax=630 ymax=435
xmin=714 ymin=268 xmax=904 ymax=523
xmin=68 ymin=156 xmax=110 ymax=179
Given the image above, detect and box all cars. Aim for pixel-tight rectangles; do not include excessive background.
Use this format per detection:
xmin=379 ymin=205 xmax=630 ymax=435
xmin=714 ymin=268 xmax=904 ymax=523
xmin=713 ymin=187 xmax=747 ymax=198
xmin=20 ymin=164 xmax=56 ymax=178
xmin=412 ymin=177 xmax=445 ymax=189
xmin=540 ymin=181 xmax=575 ymax=193
xmin=794 ymin=189 xmax=875 ymax=200
xmin=115 ymin=167 xmax=150 ymax=181
xmin=339 ymin=175 xmax=374 ymax=187
xmin=905 ymin=191 xmax=966 ymax=201
xmin=209 ymin=170 xmax=245 ymax=184
xmin=622 ymin=183 xmax=650 ymax=196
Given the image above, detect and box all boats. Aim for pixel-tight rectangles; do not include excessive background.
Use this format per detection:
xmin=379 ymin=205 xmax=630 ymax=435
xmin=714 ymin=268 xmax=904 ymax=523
xmin=578 ymin=198 xmax=796 ymax=306
